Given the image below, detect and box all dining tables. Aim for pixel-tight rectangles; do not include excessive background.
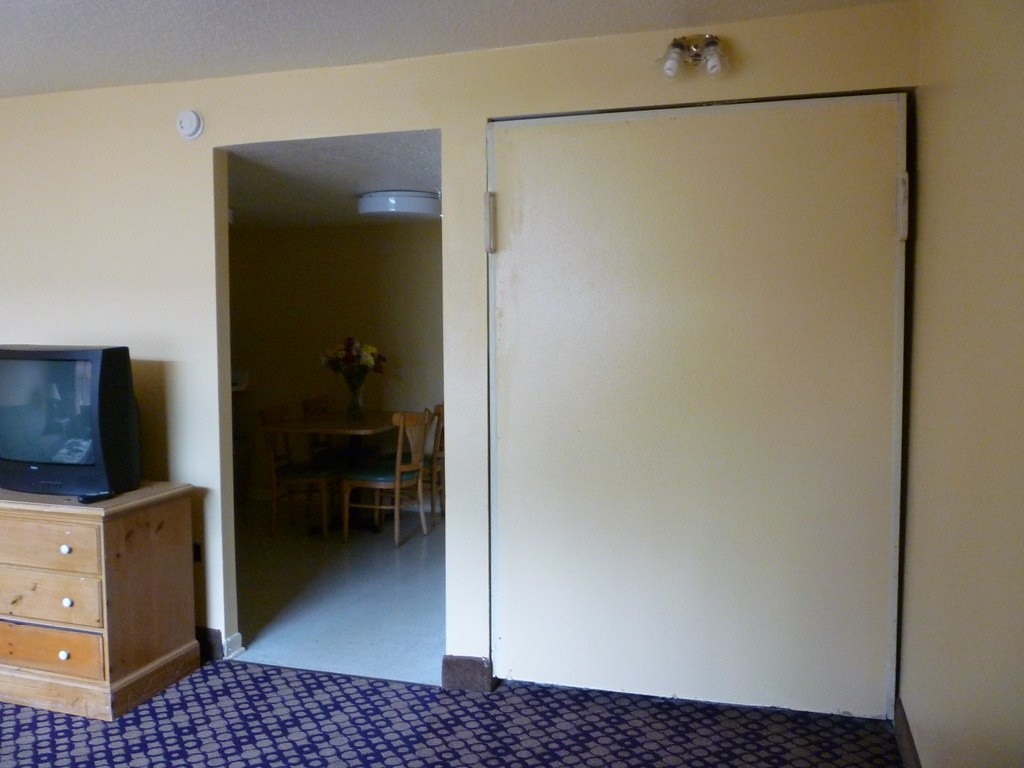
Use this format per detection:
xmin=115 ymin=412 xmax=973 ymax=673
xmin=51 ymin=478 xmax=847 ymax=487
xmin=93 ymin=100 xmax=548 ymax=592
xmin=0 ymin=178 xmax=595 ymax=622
xmin=266 ymin=408 xmax=399 ymax=538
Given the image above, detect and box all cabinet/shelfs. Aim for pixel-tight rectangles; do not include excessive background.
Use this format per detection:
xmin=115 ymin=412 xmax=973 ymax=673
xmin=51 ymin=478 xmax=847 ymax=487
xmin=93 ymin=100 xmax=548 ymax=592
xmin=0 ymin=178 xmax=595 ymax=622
xmin=0 ymin=481 xmax=200 ymax=722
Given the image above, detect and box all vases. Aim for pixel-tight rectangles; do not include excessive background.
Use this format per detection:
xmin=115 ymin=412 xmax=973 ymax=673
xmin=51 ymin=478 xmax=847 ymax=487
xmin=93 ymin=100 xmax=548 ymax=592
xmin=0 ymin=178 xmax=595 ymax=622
xmin=321 ymin=337 xmax=386 ymax=419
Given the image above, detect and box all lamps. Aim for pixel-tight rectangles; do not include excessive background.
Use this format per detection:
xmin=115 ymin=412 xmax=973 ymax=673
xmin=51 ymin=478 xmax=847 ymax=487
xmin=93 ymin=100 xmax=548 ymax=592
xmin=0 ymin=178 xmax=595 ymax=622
xmin=664 ymin=33 xmax=723 ymax=75
xmin=356 ymin=190 xmax=440 ymax=220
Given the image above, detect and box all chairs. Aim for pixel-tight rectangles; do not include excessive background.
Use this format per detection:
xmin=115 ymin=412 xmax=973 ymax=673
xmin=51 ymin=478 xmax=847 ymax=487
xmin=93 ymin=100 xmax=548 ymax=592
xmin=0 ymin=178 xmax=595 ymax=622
xmin=263 ymin=403 xmax=341 ymax=542
xmin=341 ymin=408 xmax=432 ymax=547
xmin=301 ymin=397 xmax=359 ymax=516
xmin=379 ymin=405 xmax=444 ymax=525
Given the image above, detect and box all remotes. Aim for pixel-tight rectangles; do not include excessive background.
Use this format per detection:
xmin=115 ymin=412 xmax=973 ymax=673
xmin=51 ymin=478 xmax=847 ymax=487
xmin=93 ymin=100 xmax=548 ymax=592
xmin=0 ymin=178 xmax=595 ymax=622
xmin=77 ymin=490 xmax=116 ymax=504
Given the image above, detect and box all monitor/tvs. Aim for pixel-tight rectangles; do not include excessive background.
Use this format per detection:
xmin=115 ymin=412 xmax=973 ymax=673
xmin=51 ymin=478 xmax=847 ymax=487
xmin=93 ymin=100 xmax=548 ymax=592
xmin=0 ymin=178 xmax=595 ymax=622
xmin=0 ymin=344 xmax=141 ymax=496
